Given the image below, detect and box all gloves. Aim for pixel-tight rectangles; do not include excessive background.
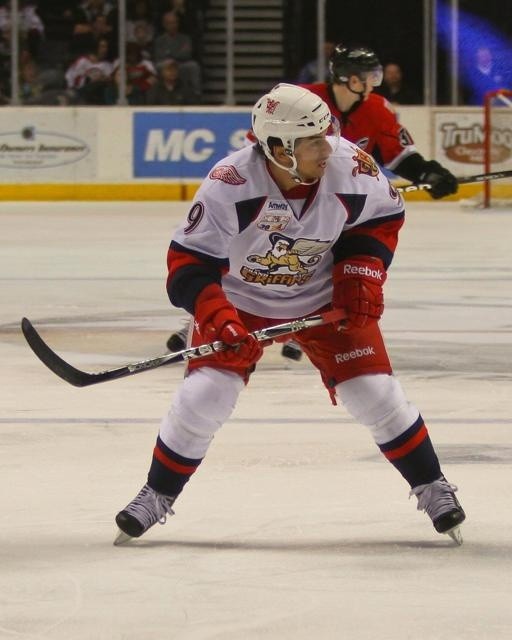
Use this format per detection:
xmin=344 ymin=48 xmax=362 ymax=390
xmin=187 ymin=280 xmax=264 ymax=370
xmin=330 ymin=257 xmax=388 ymax=338
xmin=388 ymin=148 xmax=459 ymax=200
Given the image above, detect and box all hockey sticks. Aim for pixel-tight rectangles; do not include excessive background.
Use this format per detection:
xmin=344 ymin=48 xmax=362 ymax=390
xmin=21 ymin=310 xmax=347 ymax=386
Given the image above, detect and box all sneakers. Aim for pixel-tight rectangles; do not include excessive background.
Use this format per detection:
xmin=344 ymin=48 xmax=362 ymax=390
xmin=166 ymin=323 xmax=190 ymax=352
xmin=115 ymin=481 xmax=179 ymax=540
xmin=409 ymin=478 xmax=467 ymax=536
xmin=281 ymin=341 xmax=303 ymax=361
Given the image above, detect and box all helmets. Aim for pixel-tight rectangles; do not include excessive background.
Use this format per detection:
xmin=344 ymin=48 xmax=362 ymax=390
xmin=251 ymin=80 xmax=334 ymax=153
xmin=325 ymin=43 xmax=384 ymax=85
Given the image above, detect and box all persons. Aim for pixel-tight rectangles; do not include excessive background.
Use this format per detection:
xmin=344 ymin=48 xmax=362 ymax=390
xmin=114 ymin=83 xmax=465 ymax=539
xmin=297 ymin=38 xmax=336 ymax=83
xmin=373 ymin=63 xmax=412 ymax=105
xmin=166 ymin=43 xmax=458 ymax=361
xmin=0 ymin=1 xmax=204 ymax=104
